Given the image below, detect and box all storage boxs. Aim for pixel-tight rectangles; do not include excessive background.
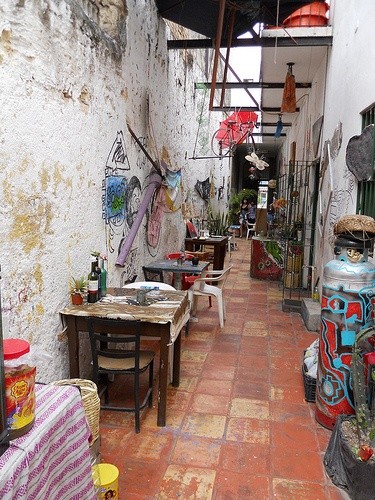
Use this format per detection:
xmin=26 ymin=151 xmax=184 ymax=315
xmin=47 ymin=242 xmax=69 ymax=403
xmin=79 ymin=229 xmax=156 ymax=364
xmin=302 ymin=349 xmax=317 ymax=402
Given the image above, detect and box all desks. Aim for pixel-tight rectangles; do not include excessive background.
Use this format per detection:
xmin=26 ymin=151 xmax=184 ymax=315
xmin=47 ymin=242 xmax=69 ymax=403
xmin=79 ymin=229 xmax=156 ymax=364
xmin=147 ymin=260 xmax=210 ymax=290
xmin=59 ymin=287 xmax=190 ymax=428
xmin=185 ymin=235 xmax=229 ymax=285
xmin=0 ymin=383 xmax=99 ymax=500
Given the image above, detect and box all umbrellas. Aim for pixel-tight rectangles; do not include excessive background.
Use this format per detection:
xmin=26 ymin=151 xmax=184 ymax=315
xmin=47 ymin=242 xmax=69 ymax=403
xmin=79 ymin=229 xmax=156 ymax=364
xmin=215 ymin=111 xmax=258 ymax=149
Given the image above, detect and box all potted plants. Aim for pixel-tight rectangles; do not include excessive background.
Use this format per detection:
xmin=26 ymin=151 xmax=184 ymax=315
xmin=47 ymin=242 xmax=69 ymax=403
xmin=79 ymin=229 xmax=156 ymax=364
xmin=69 ymin=274 xmax=91 ymax=306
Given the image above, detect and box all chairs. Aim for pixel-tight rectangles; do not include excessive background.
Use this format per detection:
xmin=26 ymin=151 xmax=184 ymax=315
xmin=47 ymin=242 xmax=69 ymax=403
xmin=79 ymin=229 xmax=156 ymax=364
xmin=87 ymin=317 xmax=156 ymax=434
xmin=166 ymin=252 xmax=212 ymax=307
xmin=108 ymin=281 xmax=177 ymax=383
xmin=187 ymin=221 xmax=198 ymax=238
xmin=142 ymin=265 xmax=164 ymax=283
xmin=188 ymin=264 xmax=233 ymax=328
xmin=245 ymin=213 xmax=256 ymax=240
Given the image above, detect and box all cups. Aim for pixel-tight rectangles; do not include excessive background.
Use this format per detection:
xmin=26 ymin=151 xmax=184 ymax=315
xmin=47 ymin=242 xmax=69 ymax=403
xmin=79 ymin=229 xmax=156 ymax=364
xmin=192 ymin=258 xmax=199 ymax=265
xmin=177 ymin=258 xmax=182 ymax=266
xmin=137 ymin=291 xmax=145 ymax=304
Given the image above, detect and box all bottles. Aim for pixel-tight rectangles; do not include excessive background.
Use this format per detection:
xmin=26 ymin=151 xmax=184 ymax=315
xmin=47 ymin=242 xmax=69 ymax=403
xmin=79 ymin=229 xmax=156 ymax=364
xmin=87 ymin=262 xmax=99 ymax=303
xmin=100 ymin=259 xmax=106 ymax=297
xmin=2 ymin=338 xmax=36 ymax=440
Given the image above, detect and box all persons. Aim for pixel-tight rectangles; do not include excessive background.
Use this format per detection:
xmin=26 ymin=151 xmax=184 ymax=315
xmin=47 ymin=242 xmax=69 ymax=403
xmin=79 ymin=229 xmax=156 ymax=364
xmin=236 ymin=198 xmax=256 ymax=237
xmin=267 ymin=204 xmax=274 ymax=224
